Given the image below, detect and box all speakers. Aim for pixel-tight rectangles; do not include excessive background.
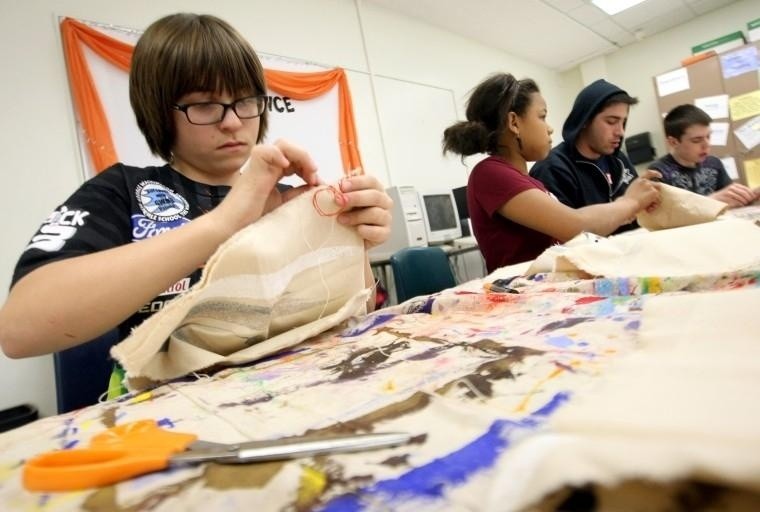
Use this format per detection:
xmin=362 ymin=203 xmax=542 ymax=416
xmin=625 ymin=131 xmax=654 ymax=164
xmin=453 ymin=186 xmax=470 ymax=219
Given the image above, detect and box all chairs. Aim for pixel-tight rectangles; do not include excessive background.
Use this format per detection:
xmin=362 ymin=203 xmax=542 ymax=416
xmin=388 ymin=245 xmax=459 ymax=305
xmin=53 ymin=327 xmax=122 ymax=415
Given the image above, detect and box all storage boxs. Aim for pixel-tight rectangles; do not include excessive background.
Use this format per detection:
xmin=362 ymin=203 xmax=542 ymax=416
xmin=653 ymin=17 xmax=760 ymax=205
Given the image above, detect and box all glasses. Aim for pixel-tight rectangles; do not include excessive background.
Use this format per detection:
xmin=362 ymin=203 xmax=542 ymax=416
xmin=173 ymin=94 xmax=269 ymax=126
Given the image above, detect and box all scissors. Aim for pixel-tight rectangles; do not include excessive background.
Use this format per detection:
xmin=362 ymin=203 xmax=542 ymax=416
xmin=21 ymin=417 xmax=412 ymax=493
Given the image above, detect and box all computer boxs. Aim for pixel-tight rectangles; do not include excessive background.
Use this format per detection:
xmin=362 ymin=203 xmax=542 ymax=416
xmin=366 ymin=184 xmax=428 ymax=262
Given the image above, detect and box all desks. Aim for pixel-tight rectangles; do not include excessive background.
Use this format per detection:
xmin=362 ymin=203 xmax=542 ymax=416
xmin=370 ymin=241 xmax=488 ymax=297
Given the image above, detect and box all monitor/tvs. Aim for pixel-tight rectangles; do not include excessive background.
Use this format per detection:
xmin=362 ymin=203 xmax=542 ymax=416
xmin=417 ymin=187 xmax=463 ymax=246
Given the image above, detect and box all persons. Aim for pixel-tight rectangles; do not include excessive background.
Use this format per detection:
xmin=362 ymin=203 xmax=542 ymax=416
xmin=646 ymin=104 xmax=760 ymax=211
xmin=527 ymin=76 xmax=647 ymax=238
xmin=441 ymin=72 xmax=671 ymax=277
xmin=0 ymin=11 xmax=394 ymax=413
